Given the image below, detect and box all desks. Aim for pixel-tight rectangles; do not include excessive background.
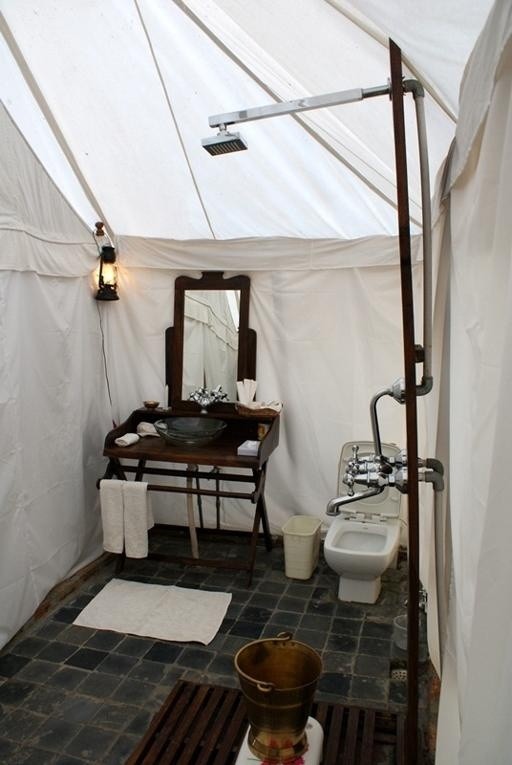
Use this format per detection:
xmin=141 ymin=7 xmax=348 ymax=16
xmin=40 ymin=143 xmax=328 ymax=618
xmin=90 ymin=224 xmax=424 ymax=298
xmin=96 ymin=403 xmax=283 ymax=587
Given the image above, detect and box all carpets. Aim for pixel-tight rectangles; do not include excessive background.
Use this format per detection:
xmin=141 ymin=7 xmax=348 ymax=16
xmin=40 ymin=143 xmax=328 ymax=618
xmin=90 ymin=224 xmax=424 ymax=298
xmin=69 ymin=578 xmax=234 ymax=646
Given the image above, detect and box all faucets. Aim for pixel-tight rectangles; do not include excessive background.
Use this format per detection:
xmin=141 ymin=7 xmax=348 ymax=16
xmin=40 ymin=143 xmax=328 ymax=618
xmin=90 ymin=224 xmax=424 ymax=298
xmin=188 ymin=391 xmax=217 ymax=409
xmin=194 ymin=384 xmax=227 ymax=400
xmin=326 ymin=486 xmax=385 ymax=518
xmin=343 ymin=472 xmax=356 ymax=497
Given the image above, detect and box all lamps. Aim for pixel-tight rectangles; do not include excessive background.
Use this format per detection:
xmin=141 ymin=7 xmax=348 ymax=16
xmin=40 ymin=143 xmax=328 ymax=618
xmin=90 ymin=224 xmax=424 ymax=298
xmin=90 ymin=221 xmax=122 ymax=302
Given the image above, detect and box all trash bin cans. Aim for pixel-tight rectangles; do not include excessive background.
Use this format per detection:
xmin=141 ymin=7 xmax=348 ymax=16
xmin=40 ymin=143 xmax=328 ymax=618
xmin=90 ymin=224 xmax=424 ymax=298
xmin=281 ymin=515 xmax=322 ymax=580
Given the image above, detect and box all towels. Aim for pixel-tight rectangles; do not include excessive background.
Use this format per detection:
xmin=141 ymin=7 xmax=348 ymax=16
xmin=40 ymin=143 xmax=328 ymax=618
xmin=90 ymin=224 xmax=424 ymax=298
xmin=97 ymin=479 xmax=125 ymax=553
xmin=115 ymin=432 xmax=140 ymax=446
xmin=122 ymin=481 xmax=157 ymax=559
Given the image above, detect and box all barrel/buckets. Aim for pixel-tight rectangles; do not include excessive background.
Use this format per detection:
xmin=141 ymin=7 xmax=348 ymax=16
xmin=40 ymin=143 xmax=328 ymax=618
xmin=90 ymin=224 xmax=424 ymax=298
xmin=232 ymin=631 xmax=325 ymax=763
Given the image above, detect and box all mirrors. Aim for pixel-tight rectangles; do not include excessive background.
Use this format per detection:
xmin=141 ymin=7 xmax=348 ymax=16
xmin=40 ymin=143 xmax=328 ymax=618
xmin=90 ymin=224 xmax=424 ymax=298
xmin=165 ymin=270 xmax=258 ymax=408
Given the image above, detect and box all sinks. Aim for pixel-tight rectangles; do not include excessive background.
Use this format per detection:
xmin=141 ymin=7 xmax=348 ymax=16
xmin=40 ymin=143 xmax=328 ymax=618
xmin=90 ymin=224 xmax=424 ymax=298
xmin=155 ymin=415 xmax=230 ymax=445
xmin=323 ymin=440 xmax=402 ymax=603
